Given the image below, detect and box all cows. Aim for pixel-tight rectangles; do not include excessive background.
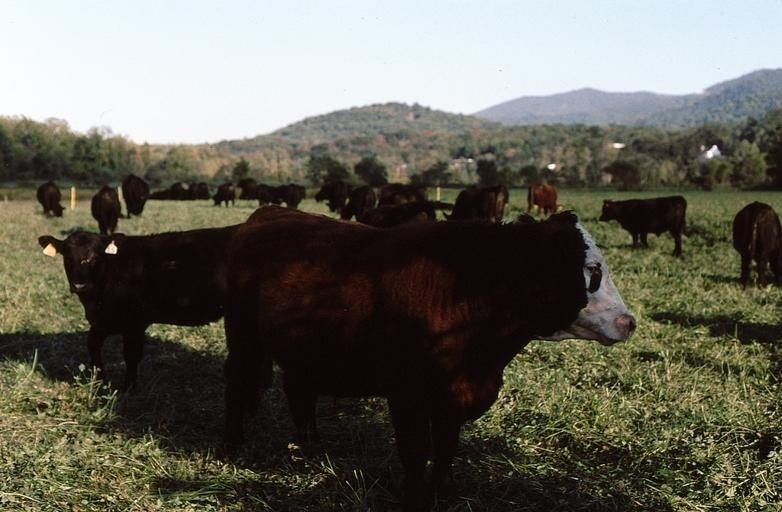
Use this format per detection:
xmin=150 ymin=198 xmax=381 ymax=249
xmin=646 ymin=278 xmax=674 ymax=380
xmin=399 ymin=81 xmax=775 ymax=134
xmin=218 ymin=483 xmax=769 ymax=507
xmin=733 ymin=200 xmax=782 ymax=291
xmin=122 ymin=173 xmax=149 ymax=218
xmin=527 ymin=181 xmax=565 ymax=217
xmin=90 ymin=184 xmax=124 ymax=236
xmin=212 ymin=178 xmax=510 ymax=224
xmin=36 ymin=180 xmax=65 ymax=217
xmin=599 ymin=195 xmax=690 ymax=258
xmin=37 ymin=222 xmax=247 ymax=396
xmin=215 ymin=203 xmax=638 ymax=512
